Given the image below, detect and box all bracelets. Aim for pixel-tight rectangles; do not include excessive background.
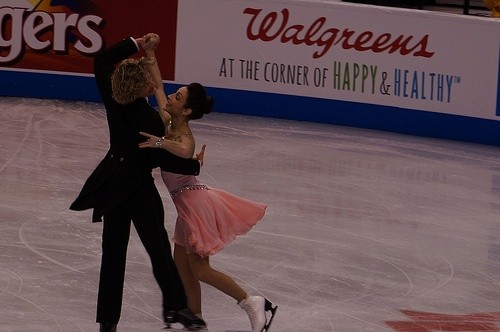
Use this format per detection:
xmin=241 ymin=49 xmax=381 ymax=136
xmin=156 ymin=136 xmax=165 ymax=148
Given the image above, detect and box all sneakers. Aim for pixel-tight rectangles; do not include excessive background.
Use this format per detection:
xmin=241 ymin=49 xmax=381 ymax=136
xmin=238 ymin=295 xmax=278 ymax=332
xmin=163 ymin=307 xmax=207 ymax=331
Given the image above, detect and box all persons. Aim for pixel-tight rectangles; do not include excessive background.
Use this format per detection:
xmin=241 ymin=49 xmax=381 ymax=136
xmin=68 ymin=32 xmax=206 ymax=331
xmin=139 ymin=33 xmax=280 ymax=331
